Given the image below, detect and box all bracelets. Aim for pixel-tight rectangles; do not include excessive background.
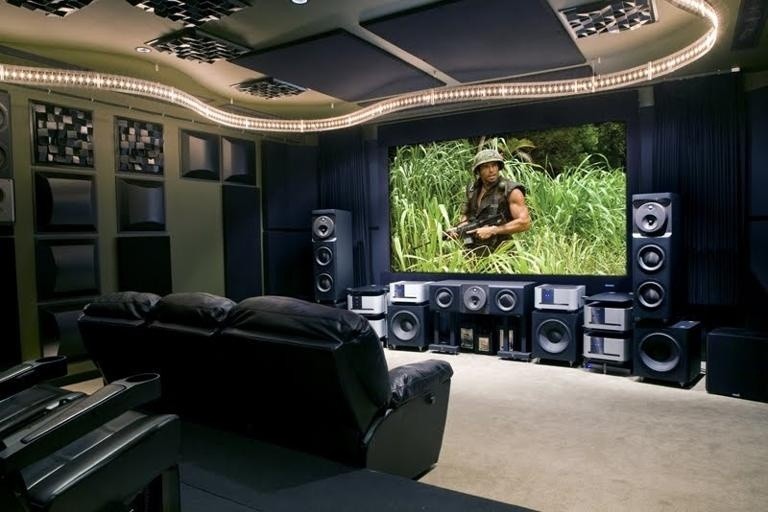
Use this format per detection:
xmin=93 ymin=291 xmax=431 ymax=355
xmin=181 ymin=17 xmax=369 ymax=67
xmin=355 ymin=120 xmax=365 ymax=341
xmin=492 ymin=225 xmax=498 ymax=236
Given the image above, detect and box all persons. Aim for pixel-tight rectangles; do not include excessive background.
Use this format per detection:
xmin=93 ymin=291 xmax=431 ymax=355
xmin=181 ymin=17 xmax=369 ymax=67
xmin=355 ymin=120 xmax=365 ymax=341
xmin=447 ymin=150 xmax=530 ymax=273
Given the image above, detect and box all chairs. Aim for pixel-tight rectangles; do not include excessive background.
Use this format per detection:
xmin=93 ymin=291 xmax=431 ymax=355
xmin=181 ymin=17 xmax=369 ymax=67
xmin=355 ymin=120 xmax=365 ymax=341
xmin=146 ymin=292 xmax=234 ymax=430
xmin=0 ymin=355 xmax=87 ymax=447
xmin=224 ymin=291 xmax=454 ymax=480
xmin=0 ymin=372 xmax=186 ymax=512
xmin=77 ymin=292 xmax=162 ymax=415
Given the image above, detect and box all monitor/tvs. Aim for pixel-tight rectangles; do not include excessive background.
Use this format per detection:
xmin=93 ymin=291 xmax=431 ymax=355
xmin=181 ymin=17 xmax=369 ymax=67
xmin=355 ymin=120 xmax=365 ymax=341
xmin=391 ymin=120 xmax=628 ymax=280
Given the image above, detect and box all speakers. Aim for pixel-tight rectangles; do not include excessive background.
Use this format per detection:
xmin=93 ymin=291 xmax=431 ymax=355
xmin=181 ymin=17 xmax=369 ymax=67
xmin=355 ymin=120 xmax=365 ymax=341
xmin=427 ymin=284 xmax=459 ymax=355
xmin=632 ymin=321 xmax=702 ymax=386
xmin=705 ymin=327 xmax=766 ymax=403
xmin=531 ymin=307 xmax=583 ymax=368
xmin=630 ymin=192 xmax=673 ymax=325
xmin=489 ymin=281 xmax=535 ymax=362
xmin=386 ymin=304 xmax=430 ymax=351
xmin=309 ymin=208 xmax=355 ymax=303
xmin=460 ymin=282 xmax=488 ymax=355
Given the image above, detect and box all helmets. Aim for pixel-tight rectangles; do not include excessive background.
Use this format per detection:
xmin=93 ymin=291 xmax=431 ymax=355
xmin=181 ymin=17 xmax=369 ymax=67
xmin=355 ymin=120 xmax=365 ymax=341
xmin=472 ymin=149 xmax=505 ymax=169
